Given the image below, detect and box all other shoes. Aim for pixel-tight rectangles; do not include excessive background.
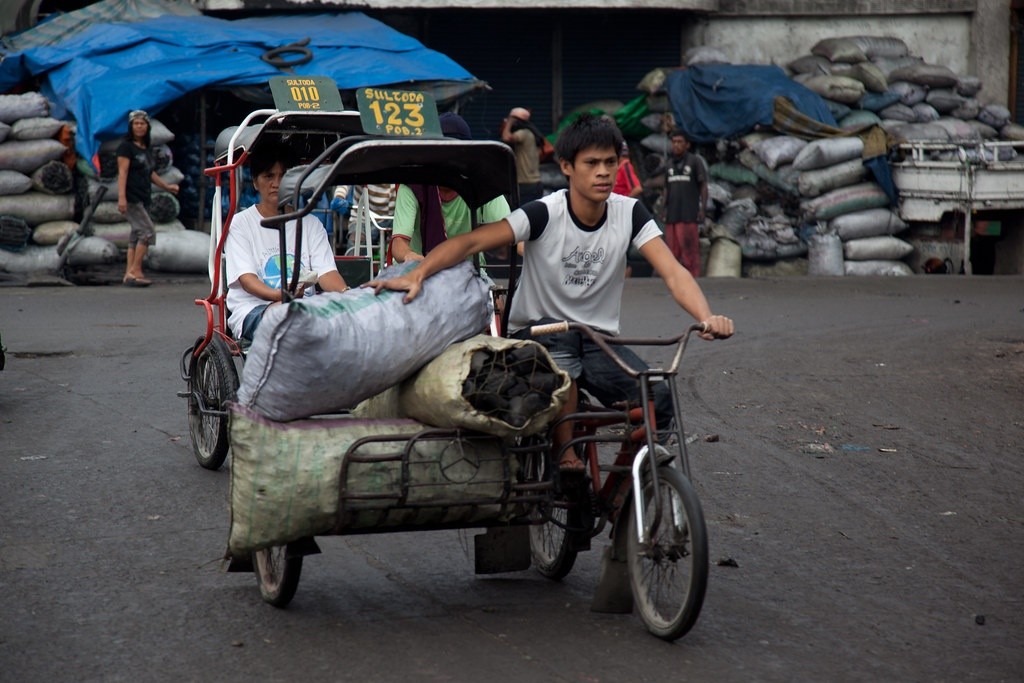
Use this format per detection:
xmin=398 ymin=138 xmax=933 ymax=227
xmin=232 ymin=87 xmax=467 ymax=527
xmin=124 ymin=274 xmax=152 ymax=287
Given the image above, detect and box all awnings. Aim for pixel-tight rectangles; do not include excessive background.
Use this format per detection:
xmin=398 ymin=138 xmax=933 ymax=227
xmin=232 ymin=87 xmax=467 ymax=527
xmin=0 ymin=0 xmax=494 ymax=184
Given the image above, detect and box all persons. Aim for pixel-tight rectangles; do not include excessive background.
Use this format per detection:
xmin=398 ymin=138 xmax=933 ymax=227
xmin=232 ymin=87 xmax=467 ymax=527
xmin=358 ymin=109 xmax=735 ymax=538
xmin=626 ymin=127 xmax=710 ymax=278
xmin=116 ymin=108 xmax=179 ymax=288
xmin=607 ymin=141 xmax=643 ymax=277
xmin=385 ymin=111 xmax=526 ymax=338
xmin=333 ymin=184 xmax=400 ymax=259
xmin=501 ymin=107 xmax=544 ymax=206
xmin=218 ymin=139 xmax=350 ymax=343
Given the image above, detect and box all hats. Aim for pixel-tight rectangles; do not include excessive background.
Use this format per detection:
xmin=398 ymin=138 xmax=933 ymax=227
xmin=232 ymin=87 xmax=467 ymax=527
xmin=438 ymin=111 xmax=473 ymax=143
xmin=510 ymin=107 xmax=530 ymax=121
xmin=128 ymin=108 xmax=150 ymax=123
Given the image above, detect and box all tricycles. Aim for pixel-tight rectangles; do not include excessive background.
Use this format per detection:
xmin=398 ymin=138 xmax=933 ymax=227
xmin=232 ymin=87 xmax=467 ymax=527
xmin=247 ymin=136 xmax=723 ymax=643
xmin=186 ymin=104 xmax=524 ymax=477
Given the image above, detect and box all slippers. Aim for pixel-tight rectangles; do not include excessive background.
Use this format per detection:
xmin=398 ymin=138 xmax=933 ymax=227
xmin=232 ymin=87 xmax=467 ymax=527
xmin=608 ymin=491 xmax=625 ymax=523
xmin=555 ymin=457 xmax=587 ymax=476
